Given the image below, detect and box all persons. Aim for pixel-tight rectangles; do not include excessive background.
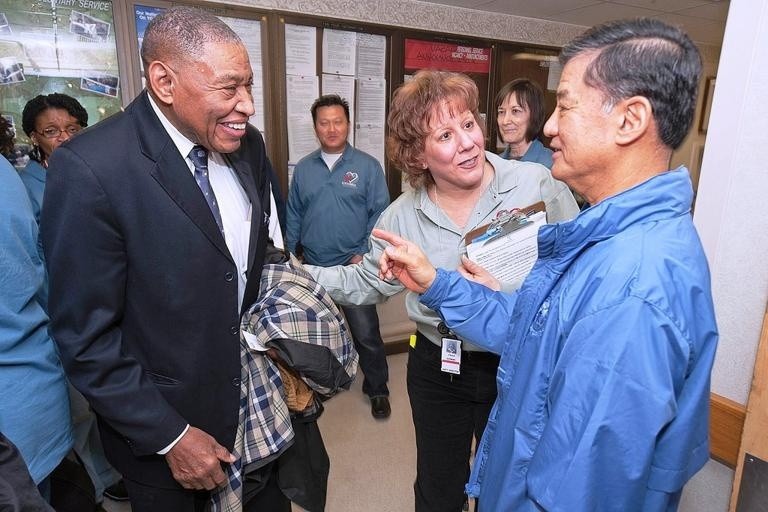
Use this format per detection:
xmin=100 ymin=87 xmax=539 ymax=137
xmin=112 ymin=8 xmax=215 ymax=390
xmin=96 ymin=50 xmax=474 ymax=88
xmin=495 ymin=76 xmax=557 ymax=173
xmin=41 ymin=7 xmax=331 ymax=512
xmin=292 ymin=68 xmax=582 ymax=512
xmin=0 ymin=154 xmax=76 ymax=510
xmin=370 ymin=17 xmax=718 ymax=512
xmin=287 ymin=91 xmax=391 ymax=418
xmin=22 ymin=79 xmax=132 ymax=511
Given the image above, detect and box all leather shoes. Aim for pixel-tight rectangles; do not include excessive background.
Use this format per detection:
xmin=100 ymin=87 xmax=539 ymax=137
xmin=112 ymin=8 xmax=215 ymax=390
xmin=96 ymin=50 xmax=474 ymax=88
xmin=370 ymin=395 xmax=391 ymax=418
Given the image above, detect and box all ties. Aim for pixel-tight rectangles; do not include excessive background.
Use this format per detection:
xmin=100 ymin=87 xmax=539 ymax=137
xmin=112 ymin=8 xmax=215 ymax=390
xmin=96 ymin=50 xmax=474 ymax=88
xmin=187 ymin=144 xmax=227 ymax=246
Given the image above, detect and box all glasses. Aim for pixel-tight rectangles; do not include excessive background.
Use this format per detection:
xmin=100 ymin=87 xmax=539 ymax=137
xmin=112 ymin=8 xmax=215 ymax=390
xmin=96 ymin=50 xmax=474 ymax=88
xmin=33 ymin=124 xmax=82 ymax=139
